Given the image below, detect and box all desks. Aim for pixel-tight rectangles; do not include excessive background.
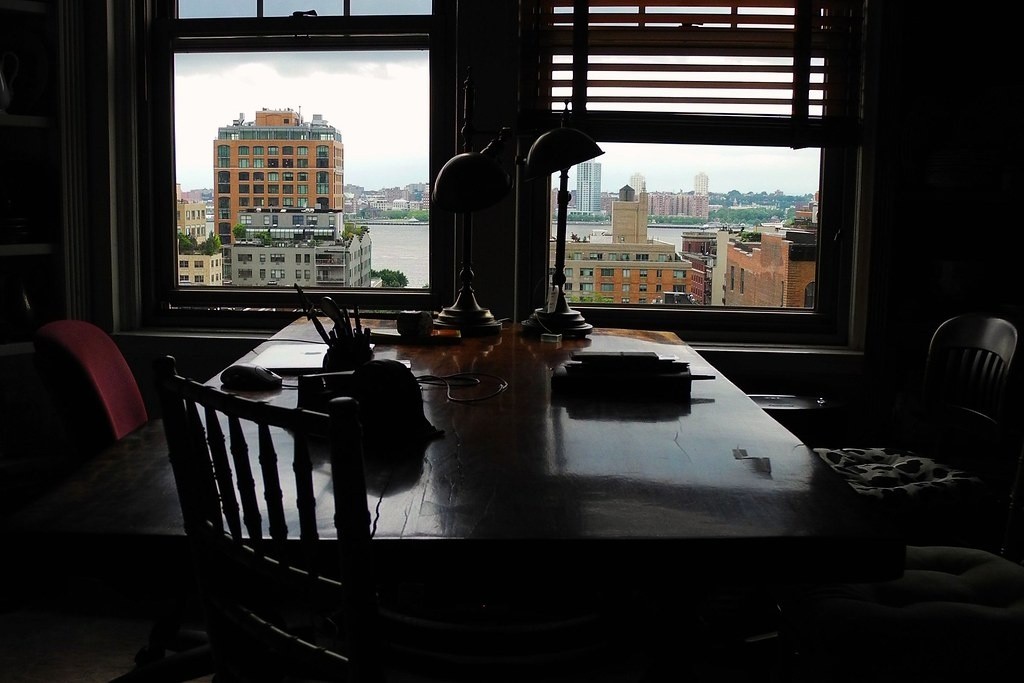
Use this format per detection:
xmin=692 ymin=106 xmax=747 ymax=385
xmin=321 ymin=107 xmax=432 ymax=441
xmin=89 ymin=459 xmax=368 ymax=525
xmin=10 ymin=313 xmax=902 ymax=683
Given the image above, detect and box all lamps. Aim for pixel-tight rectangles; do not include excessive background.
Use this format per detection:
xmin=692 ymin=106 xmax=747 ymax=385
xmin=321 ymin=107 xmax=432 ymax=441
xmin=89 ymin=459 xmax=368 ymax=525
xmin=524 ymin=105 xmax=604 ymax=335
xmin=432 ymin=66 xmax=512 ymax=331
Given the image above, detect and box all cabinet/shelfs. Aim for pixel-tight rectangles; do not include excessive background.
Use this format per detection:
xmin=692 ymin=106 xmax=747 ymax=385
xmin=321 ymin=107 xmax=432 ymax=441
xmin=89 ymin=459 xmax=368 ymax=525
xmin=0 ymin=0 xmax=72 ymax=363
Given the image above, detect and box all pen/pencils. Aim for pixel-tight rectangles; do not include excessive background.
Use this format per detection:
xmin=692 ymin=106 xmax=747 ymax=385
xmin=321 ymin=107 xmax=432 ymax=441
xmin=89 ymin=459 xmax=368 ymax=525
xmin=294 ymin=283 xmax=371 ymax=350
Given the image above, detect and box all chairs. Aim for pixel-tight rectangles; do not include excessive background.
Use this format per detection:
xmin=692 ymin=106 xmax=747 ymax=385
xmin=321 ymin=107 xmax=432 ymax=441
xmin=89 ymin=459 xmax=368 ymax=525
xmin=150 ymin=352 xmax=462 ymax=683
xmin=811 ymin=314 xmax=1018 ymax=558
xmin=34 ymin=320 xmax=333 ymax=683
xmin=745 ymin=546 xmax=1024 ymax=683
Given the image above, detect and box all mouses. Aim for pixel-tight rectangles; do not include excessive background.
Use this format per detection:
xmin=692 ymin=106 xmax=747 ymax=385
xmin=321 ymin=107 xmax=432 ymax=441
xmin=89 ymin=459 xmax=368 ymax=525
xmin=221 ymin=364 xmax=282 ymax=392
